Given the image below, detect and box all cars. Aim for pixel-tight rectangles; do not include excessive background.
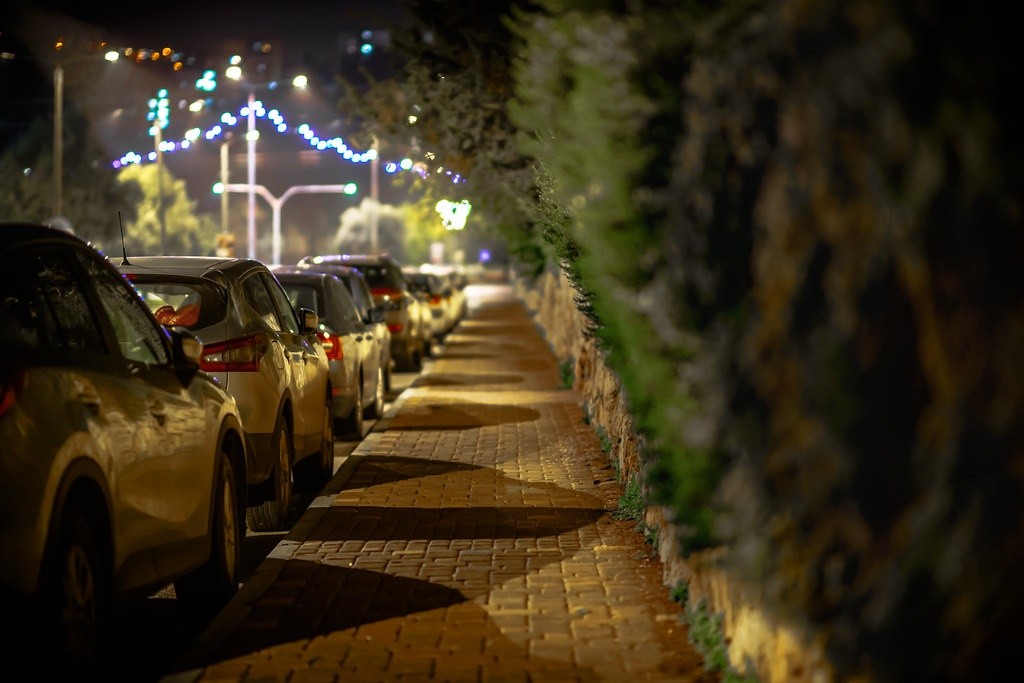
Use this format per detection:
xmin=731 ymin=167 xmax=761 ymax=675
xmin=276 ymin=272 xmax=386 ymax=440
xmin=273 ymin=264 xmax=394 ymax=398
xmin=106 ymin=257 xmax=336 ymax=533
xmin=0 ymin=217 xmax=250 ymax=667
xmin=399 ymin=270 xmax=468 ymax=354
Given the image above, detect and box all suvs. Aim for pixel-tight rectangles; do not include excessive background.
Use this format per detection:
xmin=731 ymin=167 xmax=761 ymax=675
xmin=297 ymin=251 xmax=421 ymax=371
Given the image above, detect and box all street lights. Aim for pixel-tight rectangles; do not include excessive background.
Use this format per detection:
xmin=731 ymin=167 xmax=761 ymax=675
xmin=227 ymin=61 xmax=312 ymax=265
xmin=211 ymin=181 xmax=358 ymax=266
xmin=0 ymin=48 xmax=121 ymax=231
